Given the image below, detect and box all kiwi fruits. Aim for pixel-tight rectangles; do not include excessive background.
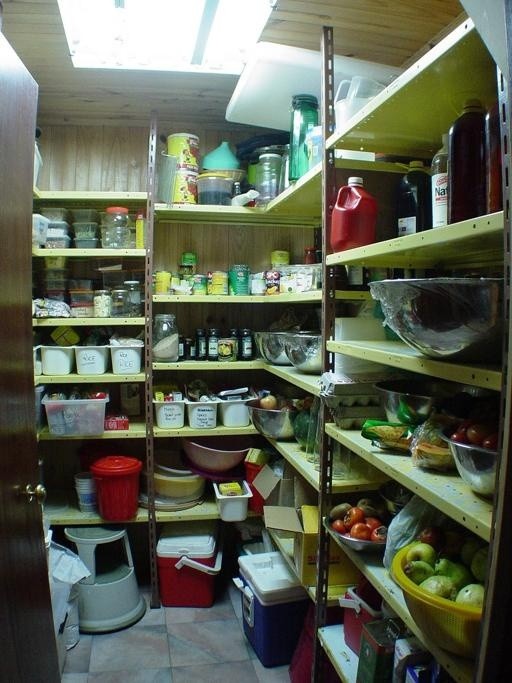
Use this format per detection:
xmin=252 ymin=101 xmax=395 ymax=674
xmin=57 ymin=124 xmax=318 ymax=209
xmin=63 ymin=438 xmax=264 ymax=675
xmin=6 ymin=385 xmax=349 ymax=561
xmin=330 ymin=502 xmax=352 ymax=521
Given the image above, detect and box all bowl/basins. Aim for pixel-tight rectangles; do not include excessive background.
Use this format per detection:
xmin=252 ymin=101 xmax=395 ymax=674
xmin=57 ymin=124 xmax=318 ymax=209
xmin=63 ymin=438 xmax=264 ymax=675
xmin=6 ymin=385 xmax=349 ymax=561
xmin=247 ymin=398 xmax=303 ymax=439
xmin=322 ymin=481 xmax=406 ymax=553
xmin=141 ymin=435 xmax=250 ymax=511
xmin=252 ymin=331 xmax=318 ymax=364
xmin=366 ymin=274 xmax=503 ymax=366
xmin=372 ymin=380 xmax=439 ymax=426
xmin=437 ymin=419 xmax=498 ymax=502
xmin=279 ymin=334 xmax=323 ymax=374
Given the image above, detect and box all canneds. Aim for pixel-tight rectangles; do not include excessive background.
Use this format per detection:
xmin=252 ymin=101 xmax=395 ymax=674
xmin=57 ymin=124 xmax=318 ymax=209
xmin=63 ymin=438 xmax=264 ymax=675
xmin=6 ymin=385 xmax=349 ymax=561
xmin=167 ymin=133 xmax=200 ymax=205
xmin=315 ymin=250 xmax=322 ymax=263
xmin=151 ymin=247 xmax=290 ymax=296
xmin=152 ymin=313 xmax=254 ymax=363
xmin=94 ymin=289 xmax=112 ymax=318
xmin=304 ymin=247 xmax=316 ymax=264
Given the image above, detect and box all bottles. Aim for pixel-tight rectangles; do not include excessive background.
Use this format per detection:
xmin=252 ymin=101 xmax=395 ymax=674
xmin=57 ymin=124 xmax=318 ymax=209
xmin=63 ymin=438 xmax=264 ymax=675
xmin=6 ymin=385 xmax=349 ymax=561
xmin=93 ymin=279 xmax=141 ymax=317
xmin=396 ymin=87 xmax=501 ymax=233
xmin=233 ymin=180 xmax=241 ymax=197
xmin=303 ymin=245 xmax=414 ymax=291
xmin=175 ymin=325 xmax=254 ymax=362
xmin=104 ymin=206 xmax=130 ymax=248
xmin=152 ymin=312 xmax=180 ymax=363
xmin=254 ymin=93 xmax=320 ymax=207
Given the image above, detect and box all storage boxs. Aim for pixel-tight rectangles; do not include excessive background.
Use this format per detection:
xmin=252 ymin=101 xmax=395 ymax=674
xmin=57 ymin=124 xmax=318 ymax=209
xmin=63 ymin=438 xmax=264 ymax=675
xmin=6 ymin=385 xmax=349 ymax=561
xmin=28 ymin=205 xmax=445 ymax=681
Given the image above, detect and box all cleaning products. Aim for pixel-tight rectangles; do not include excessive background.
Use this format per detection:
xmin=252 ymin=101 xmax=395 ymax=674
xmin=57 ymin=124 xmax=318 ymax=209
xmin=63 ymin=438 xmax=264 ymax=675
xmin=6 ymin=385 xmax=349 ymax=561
xmin=447 ymin=96 xmax=486 ymax=221
xmin=430 ymin=133 xmax=448 ymax=227
xmin=398 ymin=159 xmax=432 ymax=235
xmin=135 ymin=213 xmax=145 ymax=248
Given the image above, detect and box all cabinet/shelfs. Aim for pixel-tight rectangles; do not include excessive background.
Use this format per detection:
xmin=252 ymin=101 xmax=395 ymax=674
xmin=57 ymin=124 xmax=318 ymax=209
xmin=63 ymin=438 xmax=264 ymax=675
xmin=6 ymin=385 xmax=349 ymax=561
xmin=307 ymin=0 xmax=510 ymax=682
xmin=22 ymin=183 xmax=160 ymax=612
xmin=251 ymin=155 xmax=425 ymax=627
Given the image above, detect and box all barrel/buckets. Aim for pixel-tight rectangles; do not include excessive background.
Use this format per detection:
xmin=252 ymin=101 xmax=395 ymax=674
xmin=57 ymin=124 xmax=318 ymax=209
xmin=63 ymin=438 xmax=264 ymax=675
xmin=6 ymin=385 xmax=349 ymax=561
xmin=329 ymin=175 xmax=376 ymax=251
xmin=100 ymin=206 xmax=131 ymax=249
xmin=89 ymin=456 xmax=141 ymax=521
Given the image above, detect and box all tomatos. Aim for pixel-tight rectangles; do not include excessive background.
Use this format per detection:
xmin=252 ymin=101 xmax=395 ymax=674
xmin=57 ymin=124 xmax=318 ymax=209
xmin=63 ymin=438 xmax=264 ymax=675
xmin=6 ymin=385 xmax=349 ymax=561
xmin=451 ymin=424 xmax=499 ymax=449
xmin=331 ymin=508 xmax=387 ymax=542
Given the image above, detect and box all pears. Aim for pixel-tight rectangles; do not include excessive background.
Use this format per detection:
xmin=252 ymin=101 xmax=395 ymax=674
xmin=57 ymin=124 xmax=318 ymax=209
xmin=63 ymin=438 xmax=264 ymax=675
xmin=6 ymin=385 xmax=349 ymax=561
xmin=404 ymin=537 xmax=489 ymax=608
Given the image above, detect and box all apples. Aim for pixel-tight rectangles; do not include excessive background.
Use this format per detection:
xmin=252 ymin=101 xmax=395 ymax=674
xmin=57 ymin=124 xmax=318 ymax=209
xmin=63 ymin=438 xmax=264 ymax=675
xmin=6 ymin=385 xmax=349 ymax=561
xmin=260 ymin=394 xmax=313 ymax=412
xmin=407 ymin=527 xmax=463 ymax=566
